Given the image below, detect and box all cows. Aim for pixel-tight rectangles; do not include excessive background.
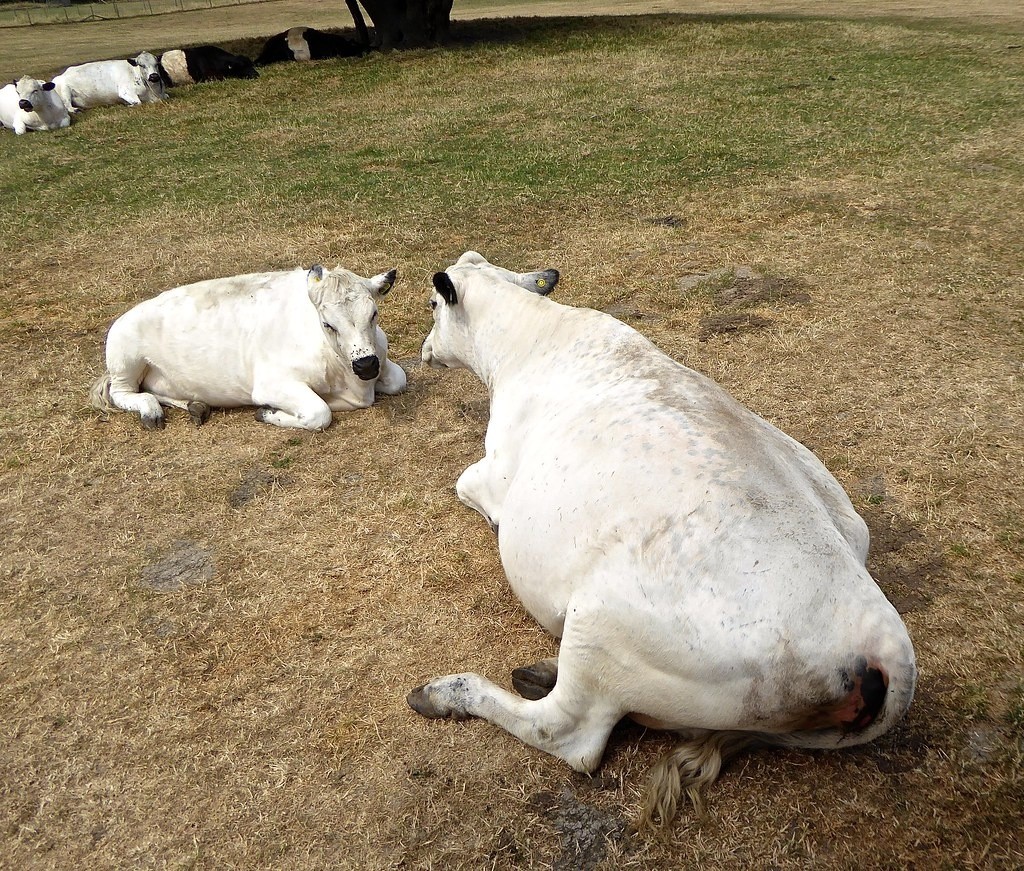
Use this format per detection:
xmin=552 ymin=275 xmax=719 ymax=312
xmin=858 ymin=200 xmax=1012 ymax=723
xmin=405 ymin=251 xmax=920 ymax=839
xmin=88 ymin=262 xmax=405 ymax=429
xmin=0 ymin=72 xmax=71 ymax=136
xmin=50 ymin=50 xmax=171 ymax=115
